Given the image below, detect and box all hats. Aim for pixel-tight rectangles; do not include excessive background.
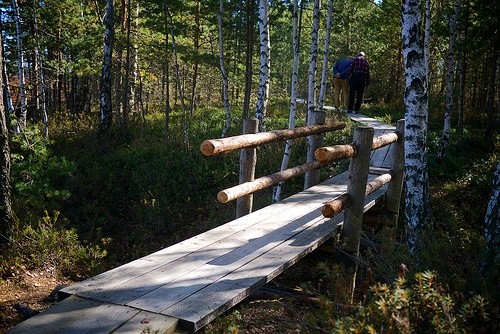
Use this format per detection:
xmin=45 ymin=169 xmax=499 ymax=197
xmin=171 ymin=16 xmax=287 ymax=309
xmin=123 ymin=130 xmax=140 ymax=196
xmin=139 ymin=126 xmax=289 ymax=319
xmin=359 ymin=51 xmax=365 ymax=56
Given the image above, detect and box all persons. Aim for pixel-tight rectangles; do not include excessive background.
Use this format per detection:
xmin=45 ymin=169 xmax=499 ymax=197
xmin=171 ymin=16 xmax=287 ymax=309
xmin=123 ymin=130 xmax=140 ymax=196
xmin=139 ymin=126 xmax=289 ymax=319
xmin=333 ymin=58 xmax=355 ymax=110
xmin=347 ymin=51 xmax=369 ymax=113
